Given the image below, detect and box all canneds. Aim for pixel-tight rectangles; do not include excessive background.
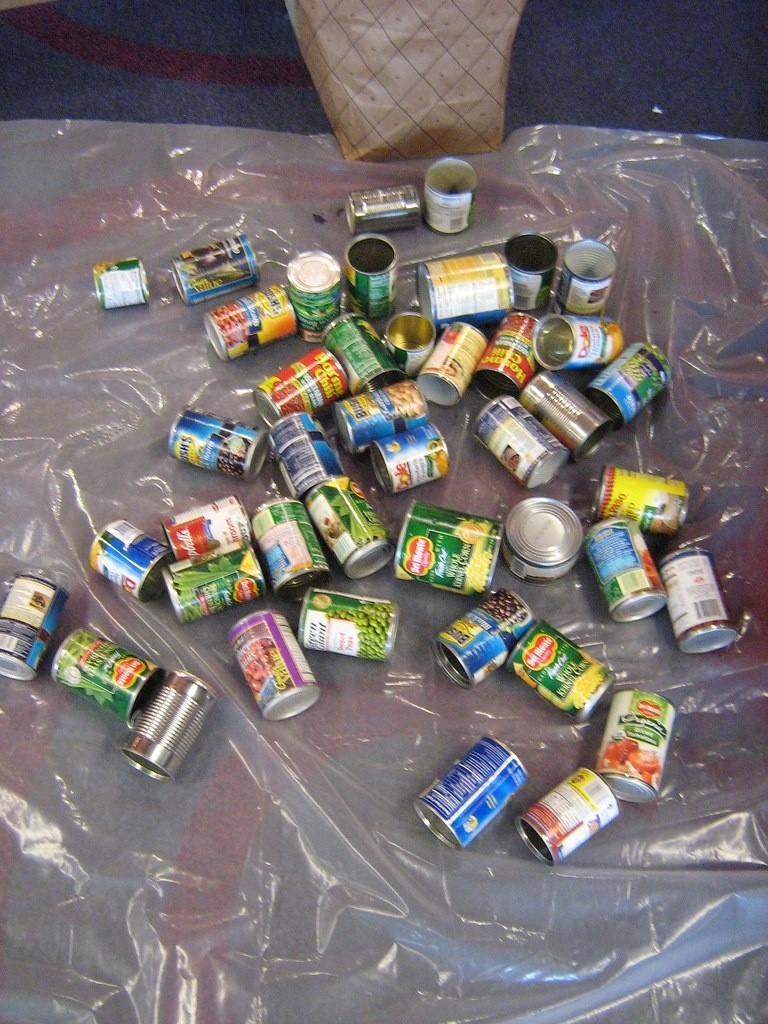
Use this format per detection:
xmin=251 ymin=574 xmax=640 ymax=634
xmin=1 ymin=157 xmax=738 ymax=866
